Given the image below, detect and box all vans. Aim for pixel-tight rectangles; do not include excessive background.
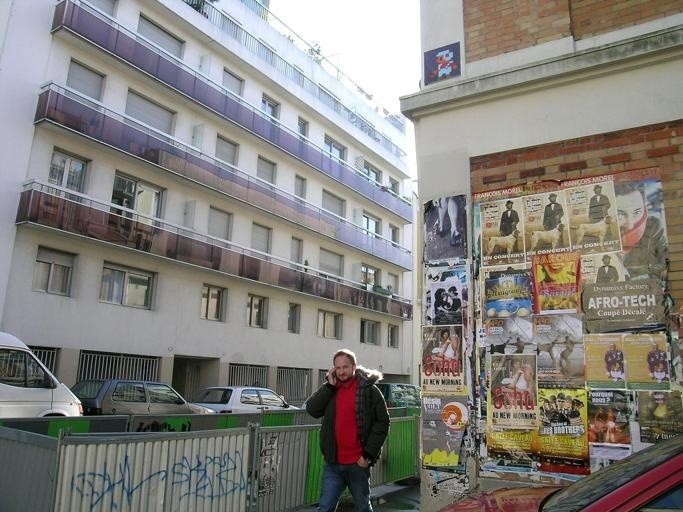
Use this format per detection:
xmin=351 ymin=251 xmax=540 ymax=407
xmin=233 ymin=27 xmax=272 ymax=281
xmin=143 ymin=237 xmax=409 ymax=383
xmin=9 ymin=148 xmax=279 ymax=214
xmin=0 ymin=329 xmax=85 ymax=422
xmin=371 ymin=381 xmax=423 ymax=407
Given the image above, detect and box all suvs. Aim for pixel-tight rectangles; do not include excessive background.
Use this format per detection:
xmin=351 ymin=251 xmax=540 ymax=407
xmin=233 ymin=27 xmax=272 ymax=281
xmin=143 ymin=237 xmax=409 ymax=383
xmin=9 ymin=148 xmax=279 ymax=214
xmin=66 ymin=375 xmax=219 ymax=418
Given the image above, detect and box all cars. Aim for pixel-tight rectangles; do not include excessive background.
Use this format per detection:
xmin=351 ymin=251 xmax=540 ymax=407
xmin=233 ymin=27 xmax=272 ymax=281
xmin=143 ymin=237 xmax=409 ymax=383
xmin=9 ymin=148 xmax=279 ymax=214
xmin=433 ymin=431 xmax=681 ymax=512
xmin=187 ymin=384 xmax=302 ymax=416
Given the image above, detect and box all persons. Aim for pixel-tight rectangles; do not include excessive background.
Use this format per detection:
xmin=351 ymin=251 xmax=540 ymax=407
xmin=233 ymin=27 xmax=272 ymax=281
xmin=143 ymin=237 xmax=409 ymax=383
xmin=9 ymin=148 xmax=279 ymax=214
xmin=424 ymin=181 xmax=668 ymax=473
xmin=303 ymin=349 xmax=391 ymax=512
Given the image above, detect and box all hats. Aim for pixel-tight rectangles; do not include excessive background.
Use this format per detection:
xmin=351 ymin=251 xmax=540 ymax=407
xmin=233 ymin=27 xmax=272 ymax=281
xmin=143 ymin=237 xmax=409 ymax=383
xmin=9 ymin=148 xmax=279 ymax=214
xmin=602 ymin=255 xmax=611 ymax=260
xmin=594 ymin=185 xmax=602 ymax=190
xmin=506 ymin=201 xmax=513 ymax=205
xmin=549 ymin=194 xmax=557 ymax=199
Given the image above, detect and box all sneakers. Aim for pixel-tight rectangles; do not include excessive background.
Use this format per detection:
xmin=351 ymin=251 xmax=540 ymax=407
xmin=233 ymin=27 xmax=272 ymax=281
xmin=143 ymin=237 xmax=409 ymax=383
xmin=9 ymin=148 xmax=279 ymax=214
xmin=450 ymin=230 xmax=462 ymax=245
xmin=436 ymin=226 xmax=444 ymax=234
